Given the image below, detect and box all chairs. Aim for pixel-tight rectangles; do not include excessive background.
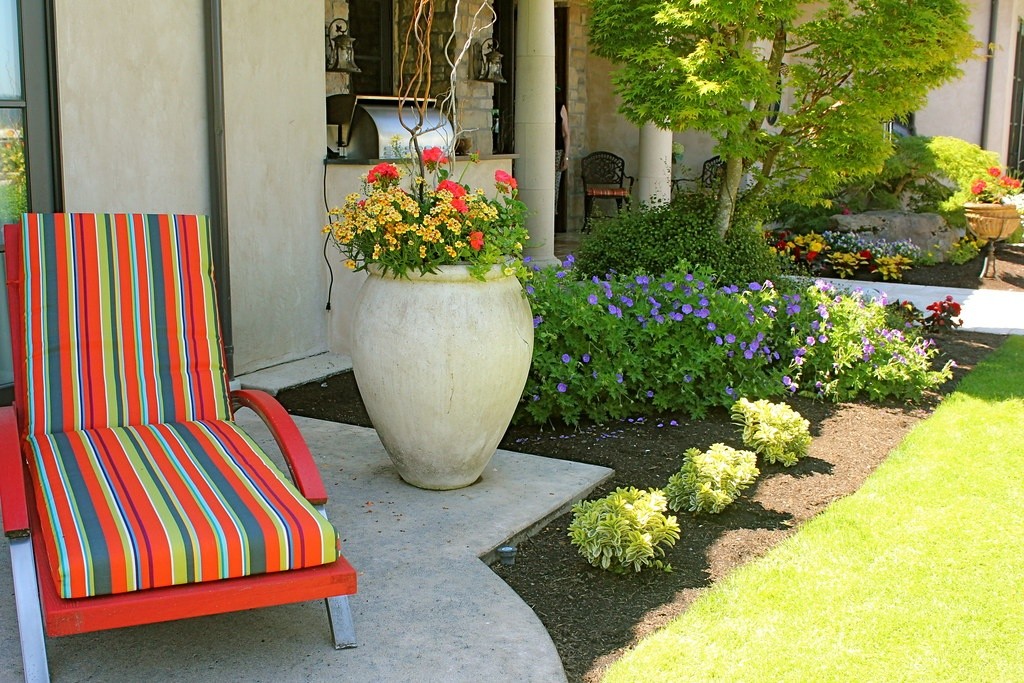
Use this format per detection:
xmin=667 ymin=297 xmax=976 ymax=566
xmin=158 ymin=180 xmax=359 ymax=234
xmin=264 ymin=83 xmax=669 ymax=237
xmin=672 ymin=154 xmax=729 ymax=195
xmin=0 ymin=213 xmax=358 ymax=683
xmin=581 ymin=152 xmax=635 ymax=232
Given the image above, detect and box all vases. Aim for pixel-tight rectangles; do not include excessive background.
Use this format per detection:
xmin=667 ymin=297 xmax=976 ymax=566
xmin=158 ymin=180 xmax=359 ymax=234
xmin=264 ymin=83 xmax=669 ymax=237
xmin=963 ymin=203 xmax=1020 ymax=239
xmin=349 ymin=263 xmax=535 ymax=490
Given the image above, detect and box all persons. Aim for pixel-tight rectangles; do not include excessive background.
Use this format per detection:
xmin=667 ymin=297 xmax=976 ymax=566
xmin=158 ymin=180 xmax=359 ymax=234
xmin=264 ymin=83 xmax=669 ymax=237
xmin=555 ymin=99 xmax=570 ymax=217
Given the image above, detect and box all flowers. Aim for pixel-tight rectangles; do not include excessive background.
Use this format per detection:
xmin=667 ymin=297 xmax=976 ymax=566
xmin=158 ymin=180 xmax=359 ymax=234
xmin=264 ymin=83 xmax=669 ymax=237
xmin=322 ymin=133 xmax=549 ymax=284
xmin=971 ymin=166 xmax=1024 ymax=203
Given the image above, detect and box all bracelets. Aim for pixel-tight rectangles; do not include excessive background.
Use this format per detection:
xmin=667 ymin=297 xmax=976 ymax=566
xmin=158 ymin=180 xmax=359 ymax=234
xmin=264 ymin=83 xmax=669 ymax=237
xmin=563 ymin=157 xmax=569 ymax=161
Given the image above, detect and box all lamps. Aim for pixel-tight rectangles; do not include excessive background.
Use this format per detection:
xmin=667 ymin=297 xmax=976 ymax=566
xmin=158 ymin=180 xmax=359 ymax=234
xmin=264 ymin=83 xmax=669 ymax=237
xmin=325 ymin=18 xmax=362 ymax=74
xmin=473 ymin=37 xmax=507 ymax=84
